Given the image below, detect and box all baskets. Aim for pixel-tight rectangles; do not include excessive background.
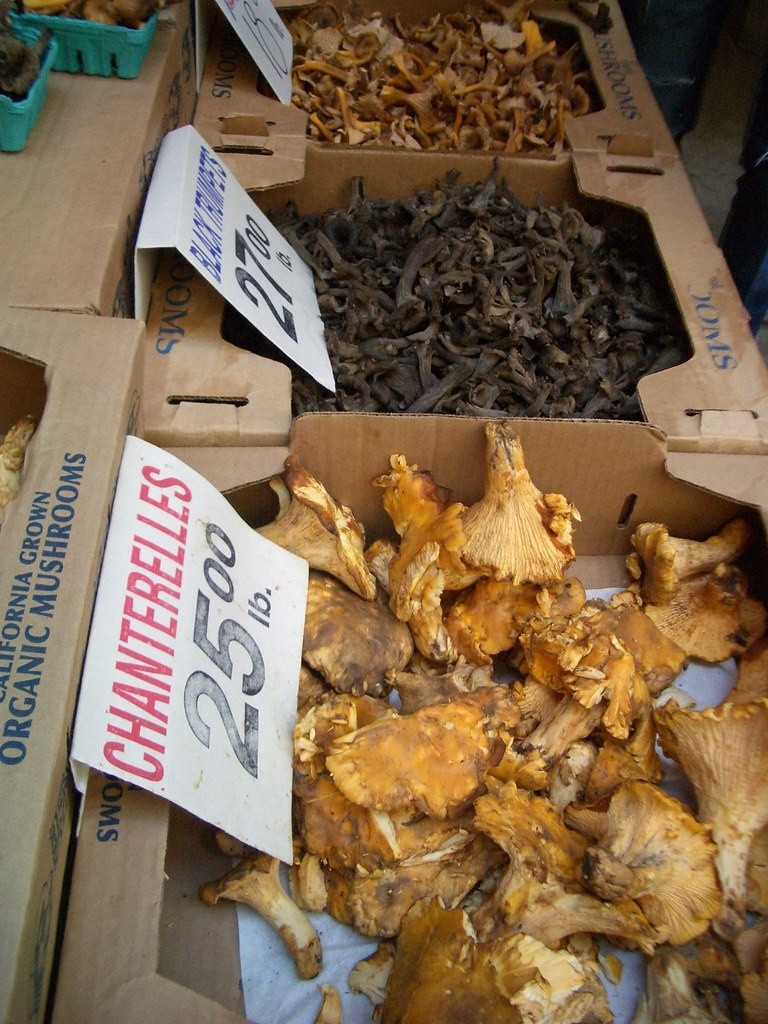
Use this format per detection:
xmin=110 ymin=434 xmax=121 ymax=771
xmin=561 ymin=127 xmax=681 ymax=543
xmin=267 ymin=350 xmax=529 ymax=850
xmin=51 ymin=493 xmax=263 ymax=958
xmin=0 ymin=22 xmax=58 ymax=151
xmin=10 ymin=9 xmax=159 ymax=80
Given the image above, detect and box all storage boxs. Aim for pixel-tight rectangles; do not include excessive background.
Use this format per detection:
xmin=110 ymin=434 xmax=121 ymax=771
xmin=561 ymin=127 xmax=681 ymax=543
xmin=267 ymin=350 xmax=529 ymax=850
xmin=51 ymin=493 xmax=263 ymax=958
xmin=131 ymin=137 xmax=768 ymax=455
xmin=51 ymin=412 xmax=768 ymax=1024
xmin=0 ymin=23 xmax=59 ymax=152
xmin=192 ymin=0 xmax=680 ymax=155
xmin=8 ymin=6 xmax=158 ymax=79
xmin=0 ymin=309 xmax=147 ymax=1024
xmin=0 ymin=0 xmax=197 ymax=319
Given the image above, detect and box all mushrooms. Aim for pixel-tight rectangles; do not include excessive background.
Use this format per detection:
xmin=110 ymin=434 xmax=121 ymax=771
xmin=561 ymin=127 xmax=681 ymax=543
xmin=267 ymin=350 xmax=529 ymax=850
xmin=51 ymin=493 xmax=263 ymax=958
xmin=0 ymin=0 xmax=161 ymax=98
xmin=227 ymin=158 xmax=694 ymax=419
xmin=196 ymin=419 xmax=768 ymax=1024
xmin=261 ymin=0 xmax=601 ymax=158
